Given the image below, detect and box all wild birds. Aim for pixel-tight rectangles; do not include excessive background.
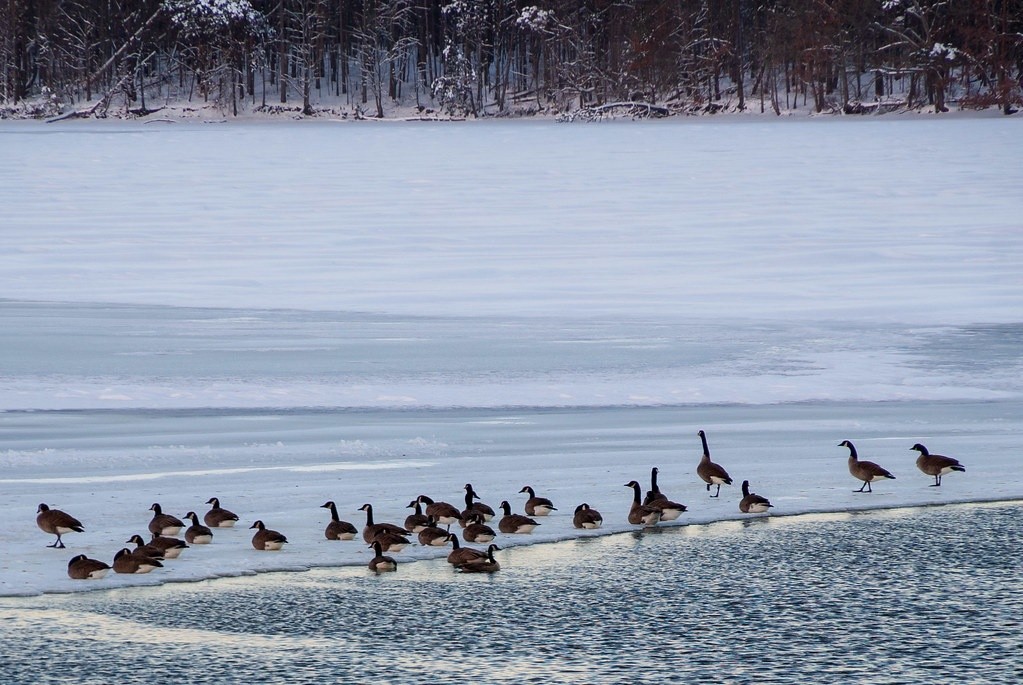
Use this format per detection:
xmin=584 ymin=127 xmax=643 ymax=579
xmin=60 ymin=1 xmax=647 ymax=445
xmin=320 ymin=501 xmax=358 ymax=540
xmin=623 ymin=480 xmax=666 ymax=526
xmin=572 ymin=503 xmax=603 ymax=529
xmin=356 ymin=484 xmax=558 ymax=573
xmin=248 ymin=520 xmax=289 ymax=550
xmin=36 ymin=503 xmax=85 ymax=548
xmin=696 ymin=430 xmax=733 ymax=498
xmin=909 ymin=443 xmax=966 ymax=487
xmin=67 ymin=498 xmax=239 ymax=580
xmin=837 ymin=440 xmax=896 ymax=493
xmin=644 ymin=491 xmax=689 ymax=521
xmin=739 ymin=480 xmax=774 ymax=513
xmin=643 ymin=467 xmax=668 ymax=506
